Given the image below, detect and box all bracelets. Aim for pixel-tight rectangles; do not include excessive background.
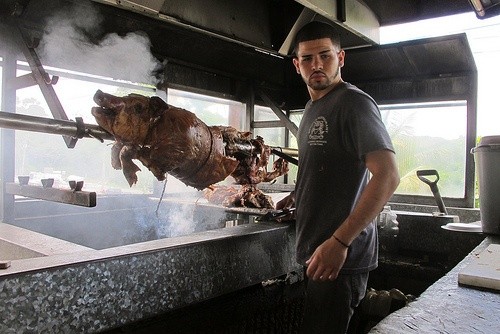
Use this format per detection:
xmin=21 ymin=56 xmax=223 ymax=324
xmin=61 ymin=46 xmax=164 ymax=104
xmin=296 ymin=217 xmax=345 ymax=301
xmin=332 ymin=235 xmax=349 ymax=248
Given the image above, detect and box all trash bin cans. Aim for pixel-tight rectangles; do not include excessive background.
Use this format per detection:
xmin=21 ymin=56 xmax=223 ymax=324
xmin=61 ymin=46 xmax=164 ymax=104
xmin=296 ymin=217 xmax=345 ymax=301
xmin=469 ymin=134 xmax=500 ymax=236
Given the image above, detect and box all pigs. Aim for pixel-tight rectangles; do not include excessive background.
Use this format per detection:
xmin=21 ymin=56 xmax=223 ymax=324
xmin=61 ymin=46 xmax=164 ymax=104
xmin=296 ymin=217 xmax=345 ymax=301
xmin=91 ymin=88 xmax=289 ymax=210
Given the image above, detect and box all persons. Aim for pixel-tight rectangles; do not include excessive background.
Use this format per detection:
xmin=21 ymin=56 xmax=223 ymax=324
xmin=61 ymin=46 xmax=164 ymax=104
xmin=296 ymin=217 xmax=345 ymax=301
xmin=274 ymin=22 xmax=400 ymax=334
